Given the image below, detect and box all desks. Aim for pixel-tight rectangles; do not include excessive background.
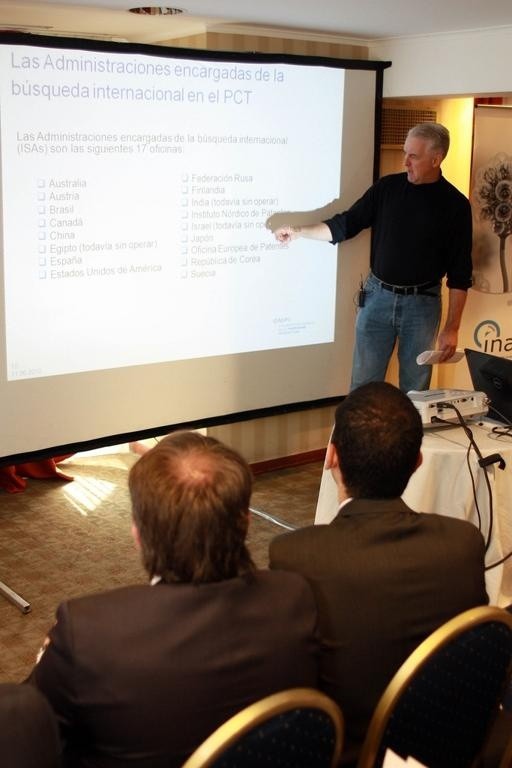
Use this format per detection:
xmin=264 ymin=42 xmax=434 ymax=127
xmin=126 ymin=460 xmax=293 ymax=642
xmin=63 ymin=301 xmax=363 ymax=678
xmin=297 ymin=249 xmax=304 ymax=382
xmin=309 ymin=410 xmax=511 ymax=612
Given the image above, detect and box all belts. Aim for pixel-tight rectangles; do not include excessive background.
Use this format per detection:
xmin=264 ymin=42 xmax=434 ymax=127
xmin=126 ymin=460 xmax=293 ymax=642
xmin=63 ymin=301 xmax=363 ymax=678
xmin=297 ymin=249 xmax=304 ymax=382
xmin=367 ymin=272 xmax=445 ymax=296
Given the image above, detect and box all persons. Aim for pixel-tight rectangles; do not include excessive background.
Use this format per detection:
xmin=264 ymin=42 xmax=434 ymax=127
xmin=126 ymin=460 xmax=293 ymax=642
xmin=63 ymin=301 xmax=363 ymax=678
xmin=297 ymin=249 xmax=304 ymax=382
xmin=1 ymin=677 xmax=67 ymax=766
xmin=270 ymin=122 xmax=474 ymax=394
xmin=32 ymin=433 xmax=318 ymax=767
xmin=271 ymin=381 xmax=487 ymax=765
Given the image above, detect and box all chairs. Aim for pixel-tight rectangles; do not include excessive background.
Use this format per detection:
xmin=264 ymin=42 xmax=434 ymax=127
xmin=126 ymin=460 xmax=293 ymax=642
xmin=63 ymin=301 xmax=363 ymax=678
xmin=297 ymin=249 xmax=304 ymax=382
xmin=353 ymin=600 xmax=511 ymax=768
xmin=178 ymin=683 xmax=352 ymax=766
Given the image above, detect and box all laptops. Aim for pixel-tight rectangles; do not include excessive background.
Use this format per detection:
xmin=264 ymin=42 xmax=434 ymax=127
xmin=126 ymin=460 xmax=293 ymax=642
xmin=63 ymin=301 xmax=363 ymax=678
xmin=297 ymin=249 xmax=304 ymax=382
xmin=464 ymin=348 xmax=512 ymax=425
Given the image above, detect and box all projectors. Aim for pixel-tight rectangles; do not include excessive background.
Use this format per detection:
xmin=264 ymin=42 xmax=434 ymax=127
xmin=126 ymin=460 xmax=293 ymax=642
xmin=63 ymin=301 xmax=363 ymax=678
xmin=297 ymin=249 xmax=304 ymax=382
xmin=405 ymin=389 xmax=489 ymax=428
